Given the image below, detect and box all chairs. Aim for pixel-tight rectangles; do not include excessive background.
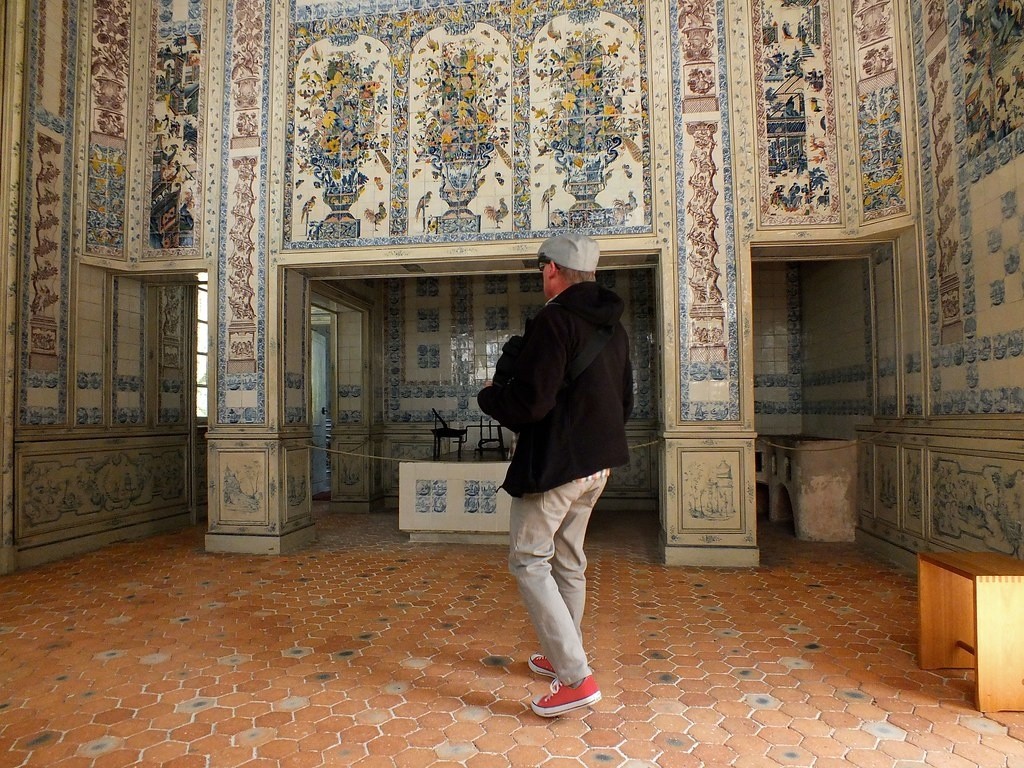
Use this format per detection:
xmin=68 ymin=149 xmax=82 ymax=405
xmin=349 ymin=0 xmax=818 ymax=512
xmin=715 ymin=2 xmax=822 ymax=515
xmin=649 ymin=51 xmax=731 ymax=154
xmin=430 ymin=408 xmax=468 ymax=461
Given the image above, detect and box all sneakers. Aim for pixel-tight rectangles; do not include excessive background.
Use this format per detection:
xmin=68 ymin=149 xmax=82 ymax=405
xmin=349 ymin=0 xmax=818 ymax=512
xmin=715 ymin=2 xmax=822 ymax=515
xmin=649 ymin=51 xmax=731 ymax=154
xmin=527 ymin=654 xmax=558 ymax=679
xmin=531 ymin=676 xmax=602 ymax=717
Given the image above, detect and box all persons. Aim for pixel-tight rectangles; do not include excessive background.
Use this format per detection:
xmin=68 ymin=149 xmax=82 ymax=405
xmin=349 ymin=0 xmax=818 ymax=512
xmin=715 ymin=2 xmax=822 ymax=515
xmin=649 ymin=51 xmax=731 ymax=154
xmin=478 ymin=232 xmax=636 ymax=718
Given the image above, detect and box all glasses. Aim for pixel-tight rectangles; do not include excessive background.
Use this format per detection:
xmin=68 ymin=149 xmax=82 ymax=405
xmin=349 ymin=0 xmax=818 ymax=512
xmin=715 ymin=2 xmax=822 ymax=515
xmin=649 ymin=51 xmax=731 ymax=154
xmin=537 ymin=259 xmax=560 ymax=271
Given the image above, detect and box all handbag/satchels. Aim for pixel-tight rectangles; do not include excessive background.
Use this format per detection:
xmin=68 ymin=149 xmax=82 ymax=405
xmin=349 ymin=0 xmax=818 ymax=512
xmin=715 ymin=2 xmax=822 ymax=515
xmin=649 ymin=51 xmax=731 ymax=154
xmin=493 ymin=335 xmax=525 ymax=386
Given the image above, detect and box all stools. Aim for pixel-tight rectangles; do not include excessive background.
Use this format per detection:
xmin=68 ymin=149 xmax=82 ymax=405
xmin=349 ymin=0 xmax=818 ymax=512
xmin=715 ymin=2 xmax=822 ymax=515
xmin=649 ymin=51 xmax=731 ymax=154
xmin=918 ymin=551 xmax=1024 ymax=713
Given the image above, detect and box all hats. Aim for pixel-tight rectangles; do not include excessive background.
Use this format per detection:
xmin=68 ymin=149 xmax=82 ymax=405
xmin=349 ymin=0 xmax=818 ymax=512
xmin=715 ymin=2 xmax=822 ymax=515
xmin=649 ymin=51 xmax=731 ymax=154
xmin=537 ymin=233 xmax=600 ymax=271
